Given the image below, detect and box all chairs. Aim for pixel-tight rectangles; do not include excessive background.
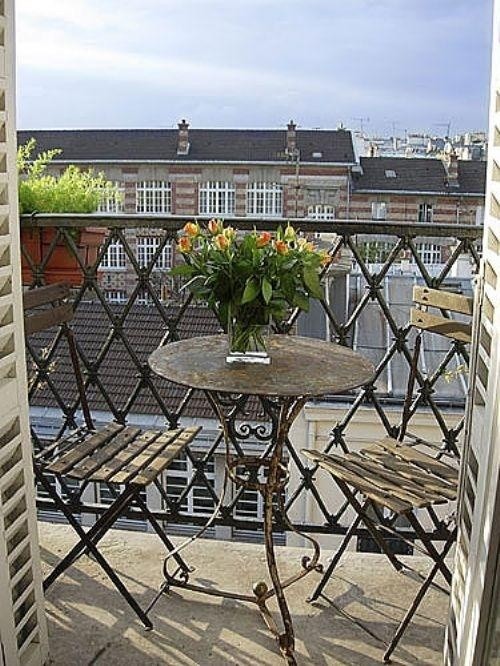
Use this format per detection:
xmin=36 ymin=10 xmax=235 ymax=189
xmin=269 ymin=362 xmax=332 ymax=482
xmin=24 ymin=282 xmax=193 ymax=631
xmin=300 ymin=286 xmax=475 ymax=664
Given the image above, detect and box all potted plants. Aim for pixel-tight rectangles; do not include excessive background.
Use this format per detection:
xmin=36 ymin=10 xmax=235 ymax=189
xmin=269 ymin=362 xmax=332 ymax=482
xmin=16 ymin=137 xmax=119 ymax=283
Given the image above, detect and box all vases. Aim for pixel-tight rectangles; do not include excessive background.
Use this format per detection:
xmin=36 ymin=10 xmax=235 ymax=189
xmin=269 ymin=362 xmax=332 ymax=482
xmin=227 ymin=324 xmax=271 ymax=363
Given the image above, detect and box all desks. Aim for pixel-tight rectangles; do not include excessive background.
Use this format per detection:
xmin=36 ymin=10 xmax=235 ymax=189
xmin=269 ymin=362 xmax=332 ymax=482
xmin=148 ymin=334 xmax=376 ymax=666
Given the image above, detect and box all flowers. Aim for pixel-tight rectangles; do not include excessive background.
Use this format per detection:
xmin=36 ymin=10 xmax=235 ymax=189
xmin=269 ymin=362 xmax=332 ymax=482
xmin=169 ymin=217 xmax=330 ymax=324
